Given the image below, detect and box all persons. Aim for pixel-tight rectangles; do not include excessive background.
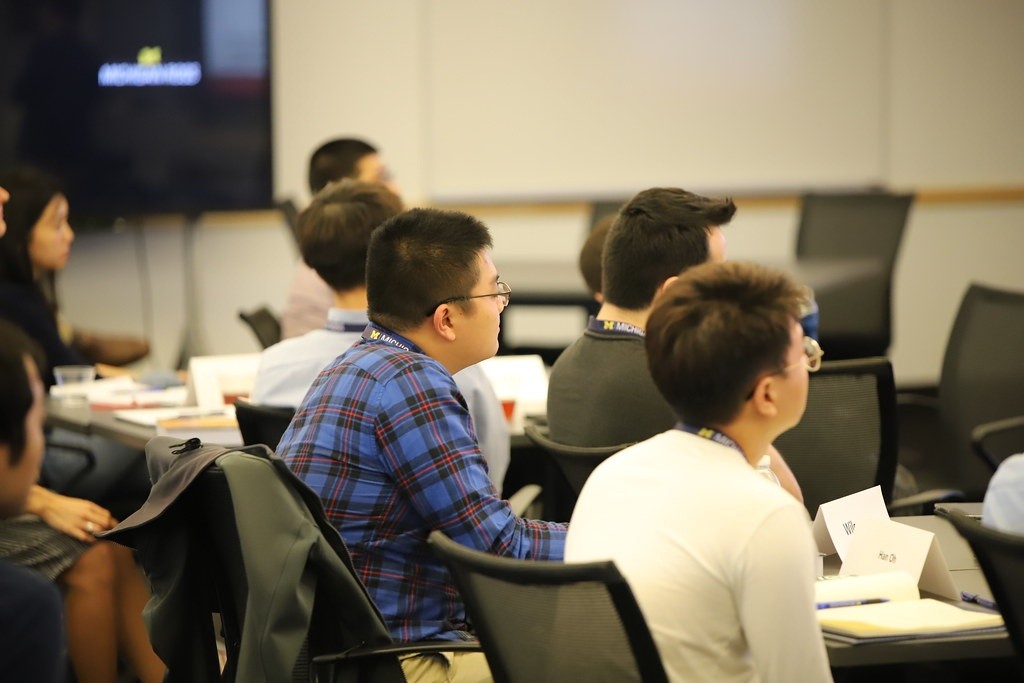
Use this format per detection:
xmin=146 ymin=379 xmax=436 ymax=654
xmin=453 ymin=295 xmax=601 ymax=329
xmin=981 ymin=452 xmax=1024 ymax=539
xmin=272 ymin=205 xmax=571 ymax=683
xmin=547 ymin=186 xmax=820 ymax=505
xmin=248 ymin=138 xmax=511 ymax=502
xmin=0 ymin=188 xmax=169 ymax=683
xmin=545 ymin=262 xmax=834 ymax=683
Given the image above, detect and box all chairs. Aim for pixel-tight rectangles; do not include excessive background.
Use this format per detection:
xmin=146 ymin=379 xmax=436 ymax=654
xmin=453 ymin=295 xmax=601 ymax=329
xmin=146 ymin=189 xmax=1024 ymax=683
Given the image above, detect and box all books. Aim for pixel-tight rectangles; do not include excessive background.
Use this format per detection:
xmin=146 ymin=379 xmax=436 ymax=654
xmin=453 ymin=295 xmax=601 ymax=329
xmin=156 ymin=415 xmax=242 ymax=449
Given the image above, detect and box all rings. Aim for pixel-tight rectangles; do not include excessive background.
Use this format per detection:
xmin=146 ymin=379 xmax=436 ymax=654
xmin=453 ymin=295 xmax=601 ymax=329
xmin=87 ymin=521 xmax=94 ymax=533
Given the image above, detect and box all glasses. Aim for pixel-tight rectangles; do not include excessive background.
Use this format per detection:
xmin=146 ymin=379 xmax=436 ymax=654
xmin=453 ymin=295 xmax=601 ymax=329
xmin=744 ymin=336 xmax=823 ymax=402
xmin=425 ymin=281 xmax=512 ymax=318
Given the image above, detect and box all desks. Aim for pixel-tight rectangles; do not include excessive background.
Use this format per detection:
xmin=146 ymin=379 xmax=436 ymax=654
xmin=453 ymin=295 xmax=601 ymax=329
xmin=42 ymin=368 xmax=245 ymax=450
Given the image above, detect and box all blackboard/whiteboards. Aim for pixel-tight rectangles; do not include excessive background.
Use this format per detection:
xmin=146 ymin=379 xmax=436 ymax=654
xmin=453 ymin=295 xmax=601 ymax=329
xmin=266 ymin=0 xmax=1023 ymax=213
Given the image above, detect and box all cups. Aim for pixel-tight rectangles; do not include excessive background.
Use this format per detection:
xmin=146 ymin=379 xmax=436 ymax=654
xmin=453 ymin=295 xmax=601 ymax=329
xmin=53 ymin=364 xmax=96 ymax=408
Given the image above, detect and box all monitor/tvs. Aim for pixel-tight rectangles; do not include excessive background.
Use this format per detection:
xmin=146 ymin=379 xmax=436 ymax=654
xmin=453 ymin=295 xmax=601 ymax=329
xmin=0 ymin=0 xmax=275 ymax=225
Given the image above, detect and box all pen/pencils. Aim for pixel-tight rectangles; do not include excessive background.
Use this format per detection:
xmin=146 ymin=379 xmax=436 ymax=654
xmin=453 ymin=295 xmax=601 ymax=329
xmin=816 ymin=599 xmax=890 ymax=610
xmin=959 ymin=591 xmax=999 ymax=611
